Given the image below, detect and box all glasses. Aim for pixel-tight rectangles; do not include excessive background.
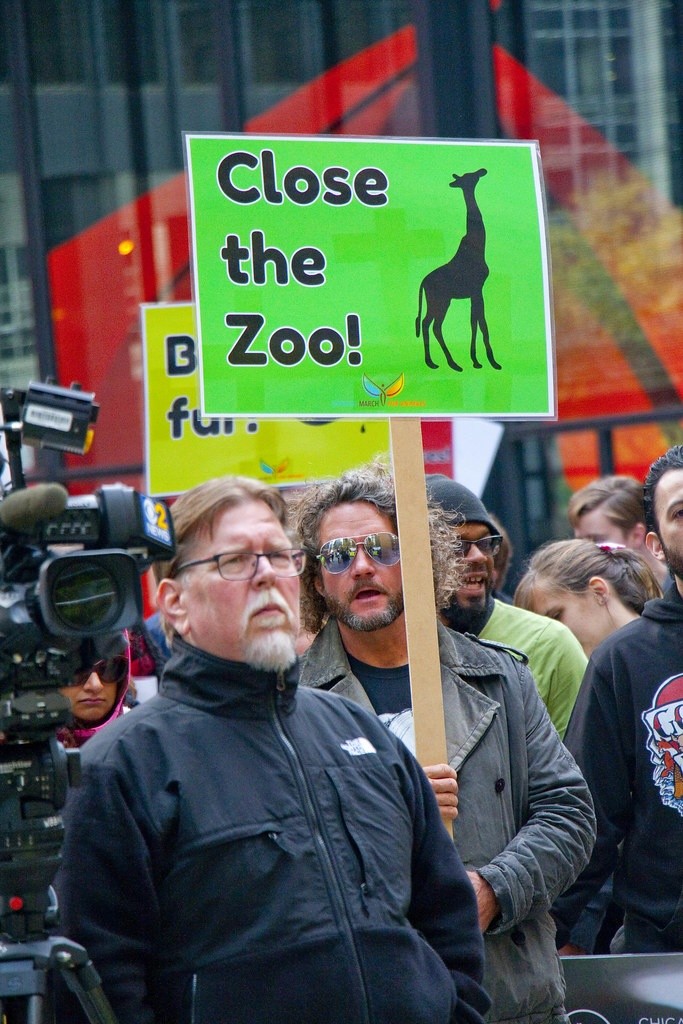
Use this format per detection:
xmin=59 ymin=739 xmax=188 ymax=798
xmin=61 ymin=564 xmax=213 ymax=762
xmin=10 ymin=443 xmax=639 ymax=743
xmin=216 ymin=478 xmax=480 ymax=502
xmin=171 ymin=550 xmax=307 ymax=581
xmin=67 ymin=656 xmax=129 ymax=687
xmin=453 ymin=534 xmax=502 ymax=558
xmin=316 ymin=532 xmax=401 ymax=575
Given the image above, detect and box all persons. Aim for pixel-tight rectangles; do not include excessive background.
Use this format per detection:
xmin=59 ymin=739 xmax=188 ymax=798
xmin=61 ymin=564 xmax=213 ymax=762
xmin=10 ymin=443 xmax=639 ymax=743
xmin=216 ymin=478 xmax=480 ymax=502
xmin=53 ymin=444 xmax=682 ymax=1024
xmin=54 ymin=476 xmax=493 ymax=1024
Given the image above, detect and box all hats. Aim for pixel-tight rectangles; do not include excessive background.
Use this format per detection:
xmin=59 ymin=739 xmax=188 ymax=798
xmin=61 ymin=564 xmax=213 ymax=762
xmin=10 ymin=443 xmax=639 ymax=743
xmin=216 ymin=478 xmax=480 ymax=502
xmin=425 ymin=470 xmax=501 ymax=540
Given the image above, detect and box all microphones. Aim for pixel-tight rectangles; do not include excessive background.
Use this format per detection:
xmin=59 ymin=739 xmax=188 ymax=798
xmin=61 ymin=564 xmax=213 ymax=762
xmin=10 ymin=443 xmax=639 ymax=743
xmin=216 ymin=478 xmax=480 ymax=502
xmin=0 ymin=485 xmax=68 ymax=529
